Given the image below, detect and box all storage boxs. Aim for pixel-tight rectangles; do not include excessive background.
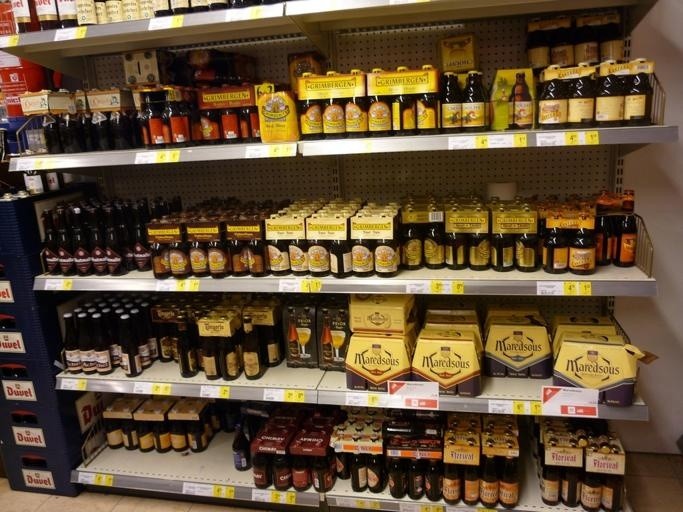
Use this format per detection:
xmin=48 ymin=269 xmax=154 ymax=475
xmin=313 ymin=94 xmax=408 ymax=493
xmin=1 ymin=365 xmax=59 ymax=410
xmin=0 ymin=312 xmax=51 ymax=364
xmin=0 ymin=194 xmax=39 ymax=261
xmin=4 ymin=455 xmax=79 ymax=498
xmin=0 ymin=412 xmax=69 ymax=453
xmin=0 ymin=264 xmax=42 ymax=310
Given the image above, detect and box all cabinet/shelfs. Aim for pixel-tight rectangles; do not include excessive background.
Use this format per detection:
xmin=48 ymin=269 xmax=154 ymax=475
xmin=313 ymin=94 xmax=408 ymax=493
xmin=0 ymin=1 xmax=679 ymax=512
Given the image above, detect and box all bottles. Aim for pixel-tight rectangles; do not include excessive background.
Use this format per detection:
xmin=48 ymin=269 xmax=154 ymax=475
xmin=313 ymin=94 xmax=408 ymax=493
xmin=77 ymin=312 xmax=97 ymax=375
xmin=42 ymin=208 xmax=61 ymax=275
xmin=139 ymin=89 xmax=165 ymax=150
xmin=490 ymin=207 xmax=516 ymax=272
xmin=291 ymin=438 xmax=313 ymax=492
xmin=193 ymin=310 xmax=205 ymax=372
xmin=185 ymin=85 xmax=200 ymax=146
xmin=424 ymin=440 xmax=443 ymax=502
xmin=574 ymin=12 xmax=601 ymax=67
xmin=163 ymin=85 xmax=191 ymax=147
xmin=480 ymin=438 xmax=499 ymax=507
xmin=601 ymin=11 xmax=624 ymax=64
xmin=199 ymin=84 xmax=221 ymax=144
xmin=109 ymin=85 xmax=131 ymax=150
xmin=168 ymin=407 xmax=189 ymax=452
xmin=322 ymin=70 xmax=346 ymax=139
xmin=116 ymin=204 xmax=138 ymax=270
xmin=403 ymin=208 xmax=424 ymax=270
xmin=368 ymin=67 xmax=392 ymax=137
xmin=320 ymin=428 xmax=337 ymax=479
xmin=242 ymin=314 xmax=264 ymax=380
xmin=388 ymin=440 xmax=408 ymax=498
xmin=461 ymin=437 xmax=480 ymax=505
xmin=149 ymin=218 xmax=173 ymax=280
xmin=58 ymin=88 xmax=83 ymax=154
xmin=288 ymin=214 xmax=310 ymax=276
xmin=422 ymin=207 xmax=445 ymax=269
xmin=226 ymin=309 xmax=244 ymax=370
xmin=187 ymin=218 xmax=211 ymax=277
xmin=515 ymin=207 xmax=537 ymax=273
xmin=367 ymin=433 xmax=385 ymax=493
xmin=351 ymin=213 xmax=376 ymax=278
xmin=63 ymin=312 xmax=82 ymax=374
xmin=73 ymin=207 xmax=91 ymax=274
xmin=228 ymin=215 xmax=250 ymax=277
xmin=537 ymin=208 xmax=548 ymax=263
xmin=550 ymin=13 xmax=574 ymax=68
xmin=102 ymin=308 xmax=121 ymax=367
xmin=499 ymin=439 xmax=522 ymax=508
xmin=90 ymin=208 xmax=107 ymax=274
xmin=286 ymin=307 xmax=300 ymax=361
xmin=105 ymin=206 xmax=126 ymax=276
xmin=153 ymin=408 xmax=172 ymax=453
xmin=445 ymin=207 xmax=468 ymax=270
xmin=141 ymin=302 xmax=159 ymax=361
xmin=467 ymin=206 xmax=491 ymax=271
xmin=195 ymin=399 xmax=215 ymax=441
xmin=207 ymin=217 xmax=229 ymax=279
xmin=252 ymin=437 xmax=272 ymax=489
xmin=509 ymin=71 xmax=532 ymax=129
xmin=624 ymin=58 xmax=653 ymax=127
xmin=89 ymin=87 xmax=113 ymax=150
xmin=76 ymin=89 xmax=92 ymax=152
xmin=538 ymin=64 xmax=567 ymax=130
xmin=238 ymin=81 xmax=261 ymax=144
xmin=568 ymin=215 xmax=597 ymax=275
xmin=175 ymin=314 xmax=198 ymax=378
xmin=541 ymin=214 xmax=569 ymax=275
xmin=154 ymin=304 xmax=174 ymax=362
xmin=92 ymin=312 xmax=113 ymax=375
xmin=407 ymin=439 xmax=425 ymax=500
xmin=122 ymin=86 xmax=142 ymax=148
xmin=104 ymin=405 xmax=124 ymax=449
xmin=273 ymin=441 xmax=292 ymax=491
xmin=345 ymin=68 xmax=369 ymax=138
xmin=232 ymin=422 xmax=251 ymax=470
xmin=169 ymin=217 xmax=193 ymax=278
xmin=120 ymin=313 xmax=143 ymax=377
xmin=351 ymin=433 xmax=367 ymax=492
xmin=57 ymin=208 xmax=75 ymax=275
xmin=171 ymin=309 xmax=186 ymax=363
xmin=266 ymin=213 xmax=292 ymax=277
xmin=415 ymin=64 xmax=440 ymax=134
xmin=218 ymin=315 xmax=240 ymax=381
xmin=442 ymin=437 xmax=462 ymax=505
xmin=528 ymin=416 xmax=625 ymax=512
xmin=121 ymin=406 xmax=140 ymax=451
xmin=299 ymin=72 xmax=322 ymax=140
xmin=136 ymin=407 xmax=155 ymax=453
xmin=132 ymin=204 xmax=153 ymax=272
xmin=186 ymin=407 xmax=209 ymax=453
xmin=478 ymin=71 xmax=490 ymax=129
xmin=567 ymin=62 xmax=596 ymax=129
xmin=130 ymin=308 xmax=152 ymax=369
xmin=335 ymin=423 xmax=351 ymax=479
xmin=201 ymin=315 xmax=222 ymax=380
xmin=320 ymin=308 xmax=333 ymax=364
xmin=596 ymin=58 xmax=624 ymax=128
xmin=461 ymin=70 xmax=485 ymax=132
xmin=440 ymin=71 xmax=462 ymax=133
xmin=373 ymin=213 xmax=398 ymax=278
xmin=391 ymin=66 xmax=416 ymax=136
xmin=526 ymin=17 xmax=550 ymax=75
xmin=219 ymin=82 xmax=241 ymax=144
xmin=261 ymin=305 xmax=282 ymax=367
xmin=247 ymin=214 xmax=266 ymax=278
xmin=595 ymin=190 xmax=613 ymax=266
xmin=0 ymin=0 xmax=282 ymax=39
xmin=307 ymin=214 xmax=330 ymax=278
xmin=612 ymin=189 xmax=638 ymax=268
xmin=329 ymin=214 xmax=353 ymax=278
xmin=41 ymin=89 xmax=64 ymax=154
xmin=311 ymin=438 xmax=334 ymax=493
